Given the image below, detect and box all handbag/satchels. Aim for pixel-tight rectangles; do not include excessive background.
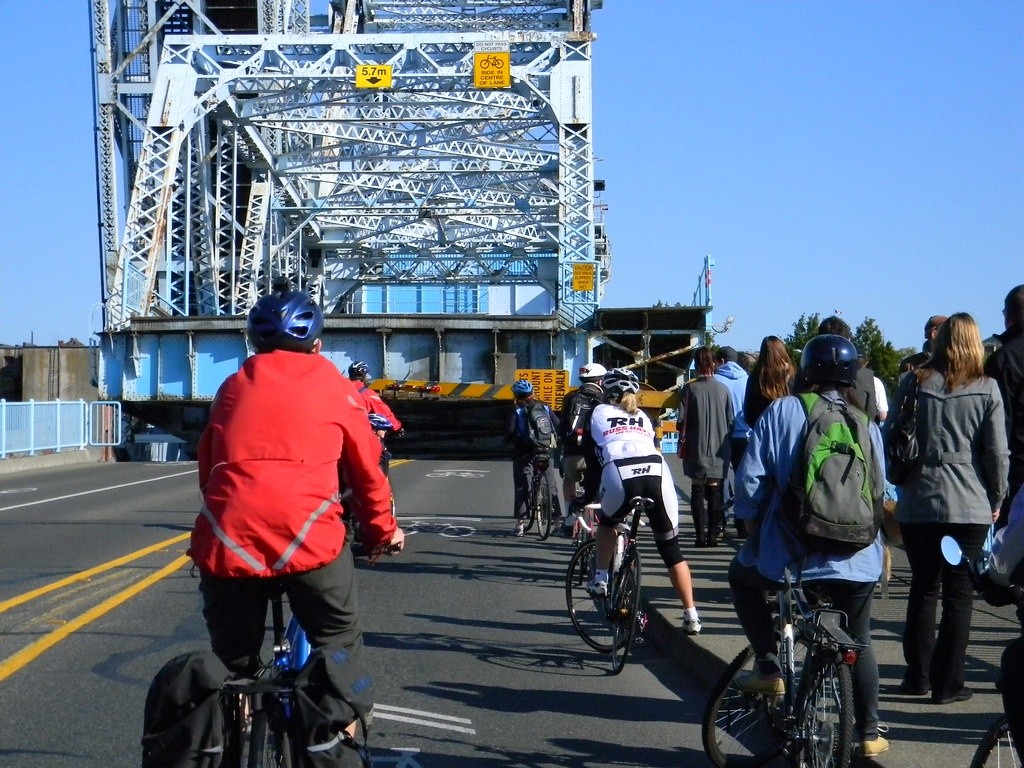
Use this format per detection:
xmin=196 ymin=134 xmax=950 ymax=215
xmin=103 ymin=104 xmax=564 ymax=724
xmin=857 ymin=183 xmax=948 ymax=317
xmin=885 ymin=367 xmax=919 ymax=485
xmin=678 ymin=443 xmax=685 ymax=457
xmin=977 ymin=523 xmax=995 ymax=575
xmin=141 ymin=643 xmax=374 ymax=768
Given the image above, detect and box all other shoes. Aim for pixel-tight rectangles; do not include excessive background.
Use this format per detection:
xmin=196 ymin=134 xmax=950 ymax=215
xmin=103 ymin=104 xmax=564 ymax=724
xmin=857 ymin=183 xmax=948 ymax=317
xmin=512 ymin=522 xmax=576 ymax=538
xmin=901 ymin=680 xmax=928 ymax=694
xmin=931 ymin=687 xmax=973 ymax=704
xmin=695 ymin=527 xmax=724 ymax=546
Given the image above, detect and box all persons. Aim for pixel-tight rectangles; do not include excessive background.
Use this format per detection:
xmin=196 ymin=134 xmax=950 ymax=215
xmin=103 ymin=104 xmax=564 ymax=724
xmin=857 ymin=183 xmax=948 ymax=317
xmin=368 ymin=414 xmax=392 ymax=475
xmin=714 ymin=346 xmax=751 ymax=540
xmin=678 ymin=348 xmax=734 ymax=546
xmin=818 ymin=317 xmax=890 ymax=421
xmin=881 ymin=283 xmax=1024 ymax=768
xmin=728 ymin=334 xmax=891 ymax=757
xmin=585 ymin=368 xmax=701 ymax=632
xmin=187 ymin=292 xmax=405 ymax=739
xmin=507 ymin=380 xmax=564 ymax=538
xmin=348 ymin=361 xmax=405 ymax=438
xmin=745 ymin=336 xmax=796 ymax=428
xmin=559 ymin=362 xmax=605 ymax=535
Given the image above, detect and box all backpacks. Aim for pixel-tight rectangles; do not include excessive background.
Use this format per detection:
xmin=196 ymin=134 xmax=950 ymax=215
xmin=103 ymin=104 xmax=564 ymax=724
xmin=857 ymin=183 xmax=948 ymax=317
xmin=785 ymin=390 xmax=884 ymax=544
xmin=563 ymin=390 xmax=600 ymax=454
xmin=522 ymin=400 xmax=557 ymax=449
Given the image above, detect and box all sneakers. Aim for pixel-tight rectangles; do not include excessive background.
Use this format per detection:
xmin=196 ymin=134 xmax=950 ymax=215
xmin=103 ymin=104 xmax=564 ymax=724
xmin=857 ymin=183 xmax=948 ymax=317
xmin=683 ymin=619 xmax=702 ymax=635
xmin=591 ymin=581 xmax=608 ymax=594
xmin=860 ymin=723 xmax=890 ymax=757
xmin=737 ymin=672 xmax=785 ymax=694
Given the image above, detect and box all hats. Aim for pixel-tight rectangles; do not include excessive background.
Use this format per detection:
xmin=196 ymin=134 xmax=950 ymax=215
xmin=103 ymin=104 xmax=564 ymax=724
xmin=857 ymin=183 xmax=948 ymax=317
xmin=993 ymin=331 xmax=1008 ymax=345
xmin=857 ymin=344 xmax=866 ymax=358
xmin=925 ymin=315 xmax=948 ymax=338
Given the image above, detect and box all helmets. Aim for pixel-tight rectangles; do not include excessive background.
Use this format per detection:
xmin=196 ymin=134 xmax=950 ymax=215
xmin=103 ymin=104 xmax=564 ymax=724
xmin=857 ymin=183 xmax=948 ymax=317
xmin=348 ymin=361 xmax=369 ymax=380
xmin=512 ymin=379 xmax=532 ymax=393
xmin=800 ymin=334 xmax=857 ymax=388
xmin=368 ymin=413 xmax=392 ymax=429
xmin=247 ymin=290 xmax=323 ymax=353
xmin=579 ymin=362 xmax=640 ymax=398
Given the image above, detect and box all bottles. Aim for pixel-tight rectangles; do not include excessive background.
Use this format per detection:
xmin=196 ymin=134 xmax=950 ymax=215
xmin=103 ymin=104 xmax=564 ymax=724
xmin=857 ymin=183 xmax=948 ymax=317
xmin=612 ymin=531 xmax=626 ymax=578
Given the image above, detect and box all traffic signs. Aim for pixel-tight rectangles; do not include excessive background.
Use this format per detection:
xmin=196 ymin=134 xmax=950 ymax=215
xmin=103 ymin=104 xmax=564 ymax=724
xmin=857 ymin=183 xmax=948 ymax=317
xmin=355 ymin=65 xmax=393 ymax=89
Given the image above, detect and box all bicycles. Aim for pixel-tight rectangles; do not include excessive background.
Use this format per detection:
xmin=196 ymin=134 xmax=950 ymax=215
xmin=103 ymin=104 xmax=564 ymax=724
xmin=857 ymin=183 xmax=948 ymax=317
xmin=524 ymin=454 xmax=552 ymax=541
xmin=700 ymin=518 xmax=871 ymax=768
xmin=970 ymin=713 xmax=1024 ymax=768
xmin=221 ymin=541 xmax=401 ymax=768
xmin=564 ymin=497 xmax=655 ymax=676
